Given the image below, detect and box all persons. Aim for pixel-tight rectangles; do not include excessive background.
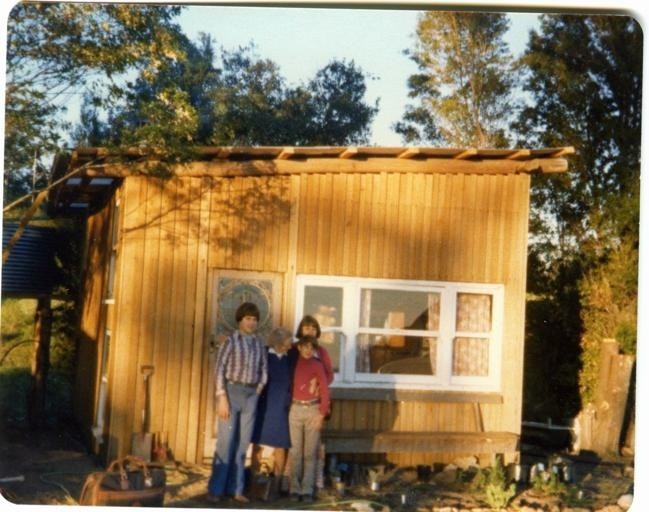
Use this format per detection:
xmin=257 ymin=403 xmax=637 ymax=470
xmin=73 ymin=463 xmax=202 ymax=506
xmin=205 ymin=301 xmax=268 ymax=504
xmin=286 ymin=333 xmax=331 ymax=504
xmin=291 ymin=315 xmax=336 ymax=492
xmin=214 ymin=328 xmax=302 ymax=502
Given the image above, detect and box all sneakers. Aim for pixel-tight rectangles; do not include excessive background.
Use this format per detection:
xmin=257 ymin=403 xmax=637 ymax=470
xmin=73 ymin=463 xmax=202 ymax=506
xmin=290 ymin=494 xmax=315 ymax=503
xmin=207 ymin=495 xmax=250 ymax=504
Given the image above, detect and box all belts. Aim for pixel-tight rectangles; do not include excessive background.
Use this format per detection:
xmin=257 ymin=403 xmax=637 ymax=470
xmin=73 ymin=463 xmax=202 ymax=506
xmin=226 ymin=380 xmax=257 ymax=388
xmin=291 ymin=399 xmax=319 ymax=406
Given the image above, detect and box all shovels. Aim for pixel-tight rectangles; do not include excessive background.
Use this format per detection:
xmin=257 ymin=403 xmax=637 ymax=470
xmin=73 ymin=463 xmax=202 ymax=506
xmin=131 ymin=365 xmax=154 ymax=462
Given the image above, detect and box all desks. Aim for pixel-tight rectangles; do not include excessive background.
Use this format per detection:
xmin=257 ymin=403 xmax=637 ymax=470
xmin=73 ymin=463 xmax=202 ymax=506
xmin=522 ymin=420 xmax=576 ymax=466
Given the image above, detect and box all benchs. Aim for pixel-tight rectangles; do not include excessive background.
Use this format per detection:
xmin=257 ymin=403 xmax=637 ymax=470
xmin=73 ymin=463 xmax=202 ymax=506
xmin=319 ymin=388 xmax=518 ymax=489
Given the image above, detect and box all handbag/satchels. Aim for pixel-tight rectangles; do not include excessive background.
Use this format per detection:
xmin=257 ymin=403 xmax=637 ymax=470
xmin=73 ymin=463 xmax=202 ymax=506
xmin=79 ymin=456 xmax=166 ymax=506
